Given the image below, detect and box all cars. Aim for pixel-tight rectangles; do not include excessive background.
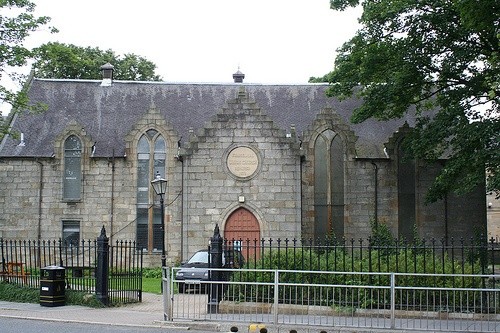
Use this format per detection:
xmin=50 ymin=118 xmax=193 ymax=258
xmin=175 ymin=248 xmax=246 ymax=294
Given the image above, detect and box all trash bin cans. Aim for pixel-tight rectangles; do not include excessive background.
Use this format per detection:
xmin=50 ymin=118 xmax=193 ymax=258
xmin=38 ymin=265 xmax=67 ymax=307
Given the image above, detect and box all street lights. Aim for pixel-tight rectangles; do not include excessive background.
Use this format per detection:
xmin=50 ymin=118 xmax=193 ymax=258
xmin=150 ymin=170 xmax=168 ymax=293
